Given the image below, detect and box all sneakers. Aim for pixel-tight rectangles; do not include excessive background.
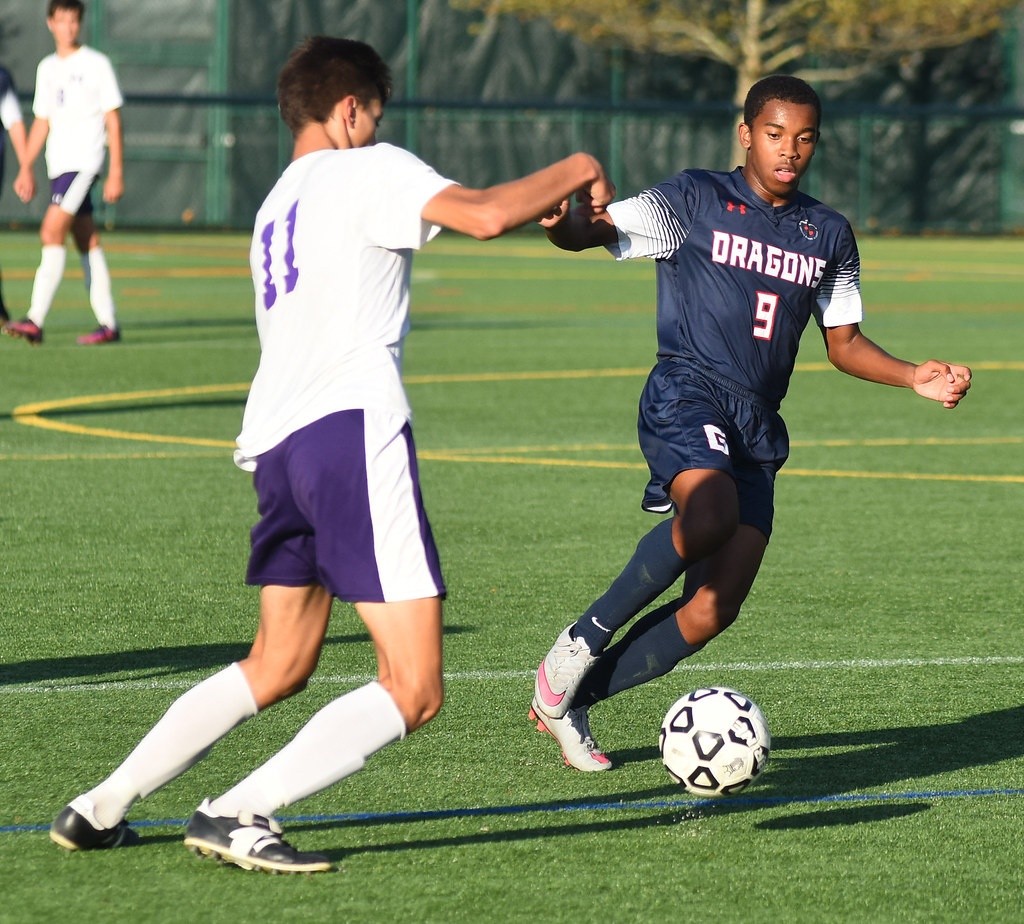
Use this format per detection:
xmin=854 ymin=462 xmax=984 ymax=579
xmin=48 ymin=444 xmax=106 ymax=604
xmin=79 ymin=326 xmax=119 ymax=347
xmin=528 ymin=696 xmax=612 ymax=771
xmin=50 ymin=796 xmax=138 ymax=850
xmin=2 ymin=318 xmax=43 ymax=344
xmin=183 ymin=796 xmax=333 ymax=874
xmin=534 ymin=622 xmax=599 ymax=721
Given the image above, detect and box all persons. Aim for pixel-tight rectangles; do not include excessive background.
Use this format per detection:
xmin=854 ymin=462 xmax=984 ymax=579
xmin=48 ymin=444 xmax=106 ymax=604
xmin=0 ymin=1 xmax=125 ymax=343
xmin=526 ymin=76 xmax=973 ymax=774
xmin=53 ymin=35 xmax=617 ymax=867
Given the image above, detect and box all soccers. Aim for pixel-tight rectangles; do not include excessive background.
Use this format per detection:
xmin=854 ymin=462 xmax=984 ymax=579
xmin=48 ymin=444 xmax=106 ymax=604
xmin=655 ymin=685 xmax=772 ymax=797
